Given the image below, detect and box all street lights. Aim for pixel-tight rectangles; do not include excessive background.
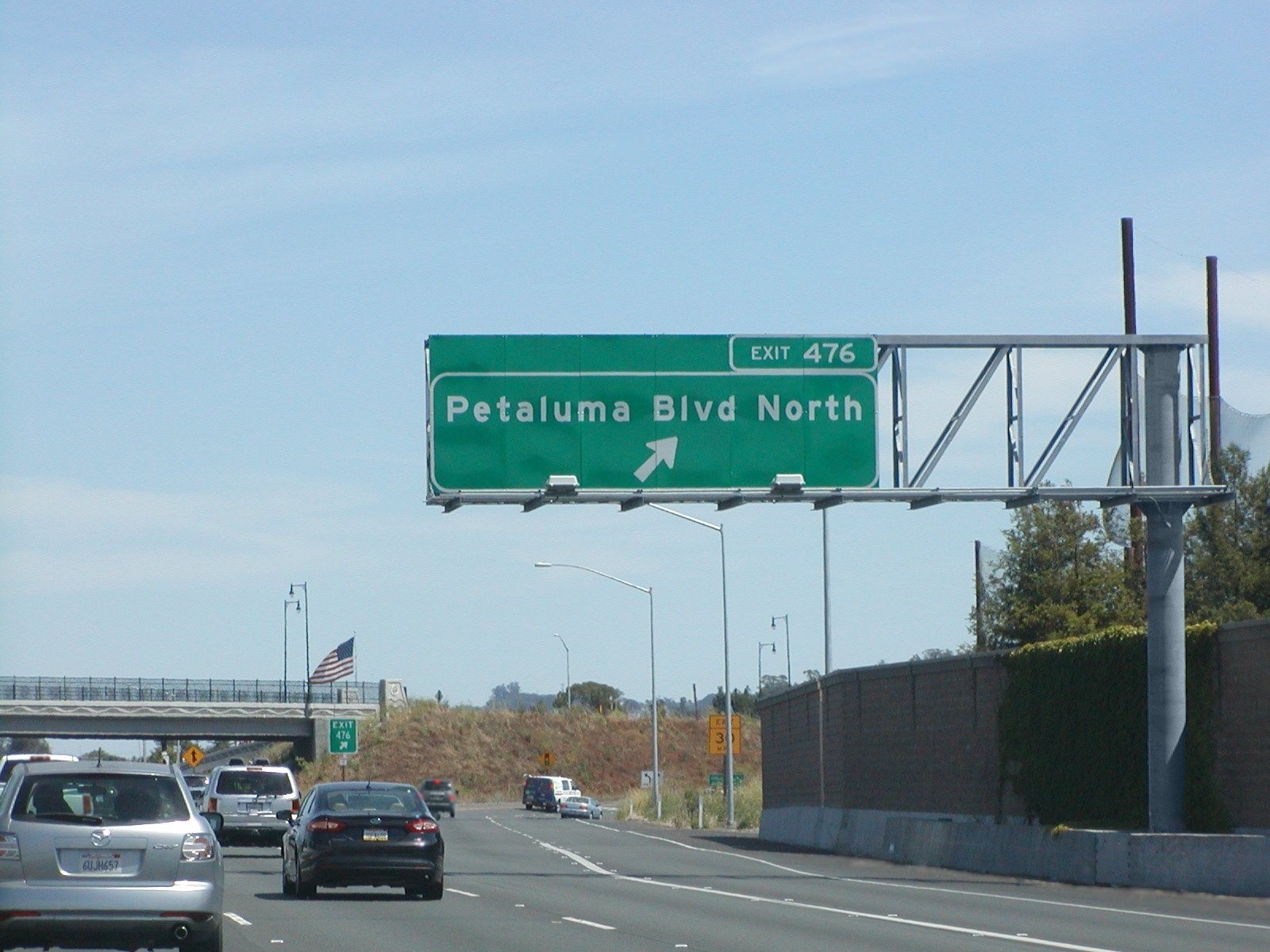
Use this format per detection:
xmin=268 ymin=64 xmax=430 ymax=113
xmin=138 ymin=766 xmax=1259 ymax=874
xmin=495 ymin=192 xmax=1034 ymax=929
xmin=290 ymin=581 xmax=313 ymax=704
xmin=770 ymin=614 xmax=791 ymax=688
xmin=534 ymin=561 xmax=662 ymax=822
xmin=759 ymin=642 xmax=776 ymax=698
xmin=553 ymin=633 xmax=570 ymax=709
xmin=285 ymin=599 xmax=301 ymax=702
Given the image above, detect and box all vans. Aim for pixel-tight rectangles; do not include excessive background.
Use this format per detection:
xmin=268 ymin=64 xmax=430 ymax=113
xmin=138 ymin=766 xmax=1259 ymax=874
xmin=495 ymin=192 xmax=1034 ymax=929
xmin=522 ymin=776 xmax=582 ymax=813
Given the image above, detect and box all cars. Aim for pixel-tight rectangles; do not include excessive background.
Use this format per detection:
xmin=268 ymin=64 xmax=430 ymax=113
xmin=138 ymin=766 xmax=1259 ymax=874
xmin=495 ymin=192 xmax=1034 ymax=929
xmin=275 ymin=773 xmax=445 ymax=899
xmin=181 ymin=772 xmax=210 ymax=803
xmin=559 ymin=795 xmax=602 ymax=820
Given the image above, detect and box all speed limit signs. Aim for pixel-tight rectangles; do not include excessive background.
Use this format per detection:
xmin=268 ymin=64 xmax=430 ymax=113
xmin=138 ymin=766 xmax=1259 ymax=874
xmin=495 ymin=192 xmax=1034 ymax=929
xmin=709 ymin=714 xmax=741 ymax=755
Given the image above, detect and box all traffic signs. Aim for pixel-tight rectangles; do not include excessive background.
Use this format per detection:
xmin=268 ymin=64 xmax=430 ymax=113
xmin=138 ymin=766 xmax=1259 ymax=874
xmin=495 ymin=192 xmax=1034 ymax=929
xmin=708 ymin=774 xmax=754 ymax=789
xmin=427 ymin=332 xmax=878 ymax=494
xmin=328 ymin=717 xmax=358 ymax=755
xmin=640 ymin=770 xmax=654 ymax=789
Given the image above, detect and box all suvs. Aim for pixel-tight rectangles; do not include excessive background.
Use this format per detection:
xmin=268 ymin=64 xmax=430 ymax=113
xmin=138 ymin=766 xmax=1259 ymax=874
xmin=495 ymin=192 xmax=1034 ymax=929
xmin=0 ymin=754 xmax=94 ymax=816
xmin=201 ymin=758 xmax=303 ymax=846
xmin=0 ymin=748 xmax=226 ymax=952
xmin=419 ymin=779 xmax=467 ymax=817
